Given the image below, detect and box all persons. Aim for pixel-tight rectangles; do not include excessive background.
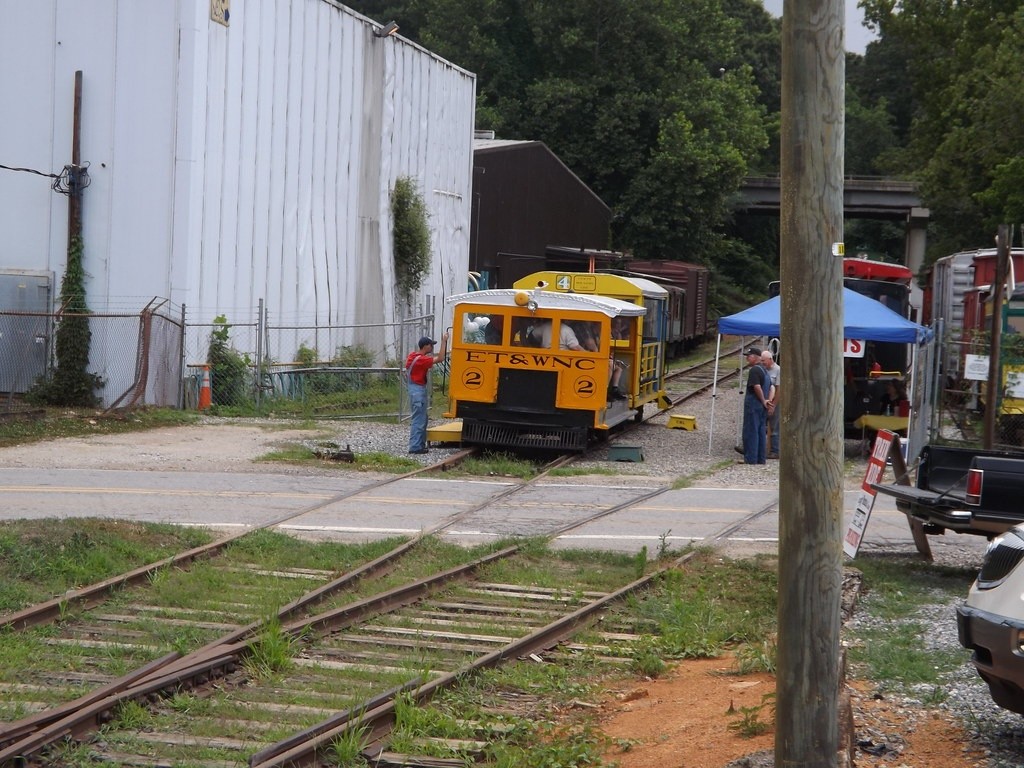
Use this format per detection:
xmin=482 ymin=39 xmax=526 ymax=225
xmin=844 ymin=357 xmax=904 ymax=465
xmin=405 ymin=333 xmax=448 ymax=454
xmin=484 ymin=315 xmax=628 ymax=402
xmin=735 ymin=349 xmax=782 ymax=463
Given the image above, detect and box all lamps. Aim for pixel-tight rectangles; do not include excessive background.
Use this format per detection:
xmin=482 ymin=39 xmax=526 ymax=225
xmin=373 ymin=20 xmax=399 ymax=38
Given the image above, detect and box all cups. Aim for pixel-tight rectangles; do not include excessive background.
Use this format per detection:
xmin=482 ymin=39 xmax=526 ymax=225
xmin=894 ymin=407 xmax=899 ymax=416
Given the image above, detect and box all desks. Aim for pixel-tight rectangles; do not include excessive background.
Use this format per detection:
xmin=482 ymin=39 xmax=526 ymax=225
xmin=853 ymin=414 xmax=909 ymax=457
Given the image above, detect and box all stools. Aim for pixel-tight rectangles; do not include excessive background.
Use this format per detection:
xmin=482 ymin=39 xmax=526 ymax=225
xmin=667 ymin=413 xmax=696 ymax=432
xmin=608 ymin=443 xmax=644 ymax=463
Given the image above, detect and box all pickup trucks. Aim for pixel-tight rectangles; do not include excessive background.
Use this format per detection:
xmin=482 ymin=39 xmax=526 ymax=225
xmin=869 ymin=444 xmax=1024 ymax=538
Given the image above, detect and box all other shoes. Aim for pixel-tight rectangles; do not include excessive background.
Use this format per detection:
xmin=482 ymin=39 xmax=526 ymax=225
xmin=607 ymin=385 xmax=626 ymax=401
xmin=409 ymin=448 xmax=429 ymax=454
xmin=766 ymin=452 xmax=780 ymax=459
xmin=734 ymin=445 xmax=745 ymax=454
xmin=737 ymin=459 xmax=747 ymax=465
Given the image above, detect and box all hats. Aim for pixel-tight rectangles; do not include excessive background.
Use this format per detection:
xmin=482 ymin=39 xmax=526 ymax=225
xmin=418 ymin=336 xmax=437 ymax=347
xmin=743 ymin=347 xmax=762 ymax=357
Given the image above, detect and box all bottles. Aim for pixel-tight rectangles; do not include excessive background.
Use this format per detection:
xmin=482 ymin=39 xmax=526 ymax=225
xmin=887 ymin=405 xmax=890 ymax=416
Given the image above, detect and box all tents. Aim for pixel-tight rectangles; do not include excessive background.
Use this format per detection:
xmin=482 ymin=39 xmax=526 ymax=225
xmin=707 ymin=288 xmax=932 ymax=468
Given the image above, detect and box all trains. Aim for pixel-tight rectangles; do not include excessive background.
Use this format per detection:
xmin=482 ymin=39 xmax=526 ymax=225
xmin=933 ymin=243 xmax=1023 ymax=441
xmin=769 ymin=258 xmax=914 ymax=439
xmin=428 ymin=258 xmax=708 ymax=456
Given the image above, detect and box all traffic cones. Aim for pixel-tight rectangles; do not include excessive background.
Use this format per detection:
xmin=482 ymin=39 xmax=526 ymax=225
xmin=196 ymin=367 xmax=212 ymax=412
